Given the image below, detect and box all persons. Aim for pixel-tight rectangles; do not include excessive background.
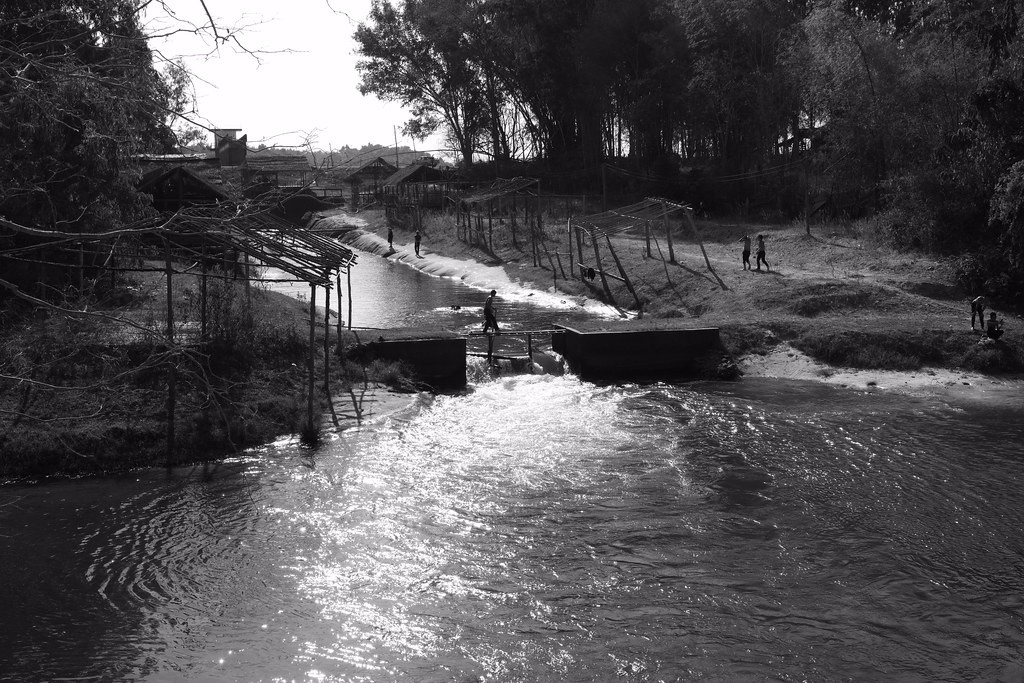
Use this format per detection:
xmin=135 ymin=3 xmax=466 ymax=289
xmin=755 ymin=235 xmax=770 ymax=271
xmin=971 ymin=294 xmax=990 ymax=331
xmin=987 ymin=312 xmax=1004 ymax=344
xmin=388 ymin=229 xmax=393 ymax=248
xmin=738 ymin=235 xmax=751 ymax=270
xmin=414 ymin=231 xmax=421 ymax=258
xmin=482 ymin=290 xmax=499 ymax=336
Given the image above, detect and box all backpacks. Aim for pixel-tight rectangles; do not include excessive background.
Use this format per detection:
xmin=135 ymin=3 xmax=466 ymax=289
xmin=587 ymin=268 xmax=596 ymax=280
